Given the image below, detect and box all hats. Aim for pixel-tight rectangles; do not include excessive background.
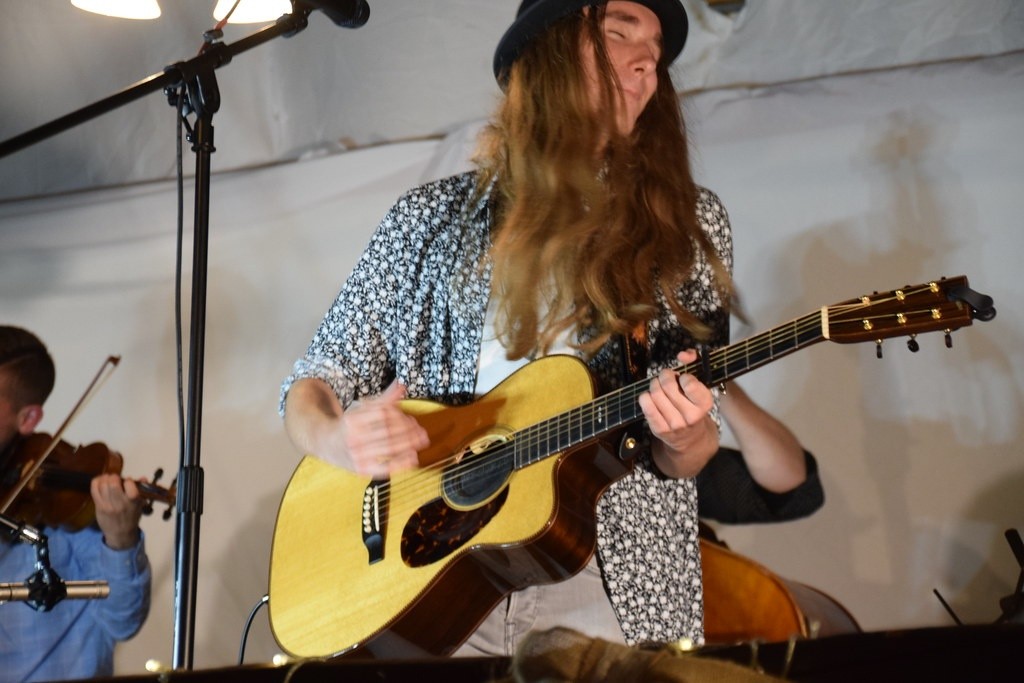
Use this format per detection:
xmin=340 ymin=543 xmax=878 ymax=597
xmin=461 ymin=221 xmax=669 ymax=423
xmin=493 ymin=0 xmax=688 ymax=96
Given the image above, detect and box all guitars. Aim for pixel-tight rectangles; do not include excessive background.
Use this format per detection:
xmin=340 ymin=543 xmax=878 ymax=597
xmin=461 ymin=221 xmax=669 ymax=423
xmin=268 ymin=276 xmax=1000 ymax=672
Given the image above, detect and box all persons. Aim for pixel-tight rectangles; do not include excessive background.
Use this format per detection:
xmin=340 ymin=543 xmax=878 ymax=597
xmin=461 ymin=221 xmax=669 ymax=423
xmin=696 ymin=376 xmax=823 ymax=524
xmin=283 ymin=1 xmax=732 ymax=678
xmin=0 ymin=320 xmax=153 ymax=683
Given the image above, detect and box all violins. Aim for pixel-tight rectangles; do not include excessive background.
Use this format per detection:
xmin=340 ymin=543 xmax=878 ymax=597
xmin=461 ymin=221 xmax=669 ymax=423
xmin=2 ymin=431 xmax=179 ymax=549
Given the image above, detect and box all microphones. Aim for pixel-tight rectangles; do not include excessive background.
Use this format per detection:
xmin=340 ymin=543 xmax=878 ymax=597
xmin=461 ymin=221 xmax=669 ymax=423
xmin=290 ymin=0 xmax=370 ymax=29
